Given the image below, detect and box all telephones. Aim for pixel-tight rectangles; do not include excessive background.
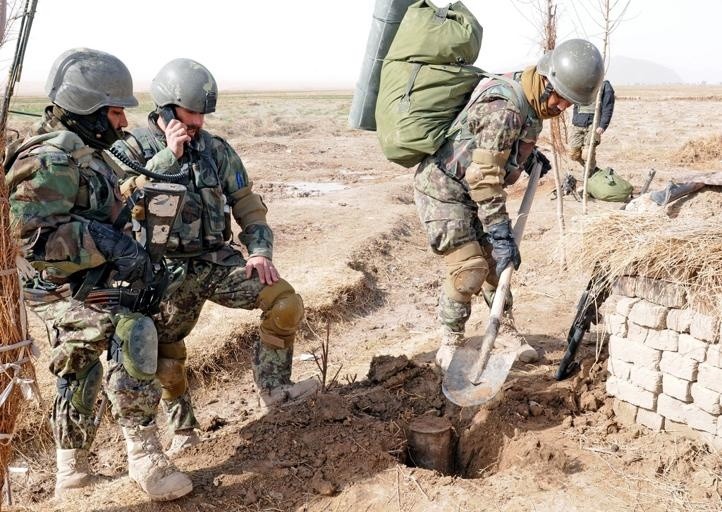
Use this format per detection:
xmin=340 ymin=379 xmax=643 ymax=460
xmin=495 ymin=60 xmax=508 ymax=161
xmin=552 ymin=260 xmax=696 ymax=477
xmin=159 ymin=106 xmax=193 ymax=154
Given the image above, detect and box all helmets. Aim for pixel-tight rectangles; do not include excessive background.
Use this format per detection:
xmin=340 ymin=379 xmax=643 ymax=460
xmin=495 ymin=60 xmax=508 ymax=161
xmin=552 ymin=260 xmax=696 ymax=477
xmin=45 ymin=47 xmax=138 ymax=116
xmin=538 ymin=40 xmax=604 ymax=106
xmin=150 ymin=58 xmax=218 ymax=114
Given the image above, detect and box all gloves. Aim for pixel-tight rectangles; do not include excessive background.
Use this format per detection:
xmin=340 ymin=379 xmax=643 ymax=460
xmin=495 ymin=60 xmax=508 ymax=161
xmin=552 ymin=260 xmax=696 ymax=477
xmin=89 ymin=219 xmax=152 ymax=286
xmin=524 ymin=149 xmax=552 ymax=179
xmin=487 ymin=220 xmax=521 ymax=277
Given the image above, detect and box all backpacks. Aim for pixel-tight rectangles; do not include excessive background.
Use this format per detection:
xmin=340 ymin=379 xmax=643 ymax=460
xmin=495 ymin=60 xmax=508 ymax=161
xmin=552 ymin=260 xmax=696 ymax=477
xmin=585 ymin=168 xmax=633 ymax=202
xmin=375 ymin=2 xmax=528 ymax=170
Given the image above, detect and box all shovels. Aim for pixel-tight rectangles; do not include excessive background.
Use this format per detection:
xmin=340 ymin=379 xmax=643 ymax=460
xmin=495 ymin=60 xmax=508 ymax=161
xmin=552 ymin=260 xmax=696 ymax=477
xmin=650 ymin=171 xmax=722 ymax=206
xmin=441 ymin=160 xmax=544 ymax=408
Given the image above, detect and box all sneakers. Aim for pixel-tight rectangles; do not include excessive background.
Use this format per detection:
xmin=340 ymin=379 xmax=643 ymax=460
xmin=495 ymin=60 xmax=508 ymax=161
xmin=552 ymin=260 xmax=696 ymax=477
xmin=436 ymin=333 xmax=466 ymax=371
xmin=122 ymin=425 xmax=194 ymax=503
xmin=492 ymin=309 xmax=538 ymax=363
xmin=56 ymin=447 xmax=113 ymax=498
xmin=165 ymin=432 xmax=200 ymax=456
xmin=259 ymin=377 xmax=320 ymax=410
